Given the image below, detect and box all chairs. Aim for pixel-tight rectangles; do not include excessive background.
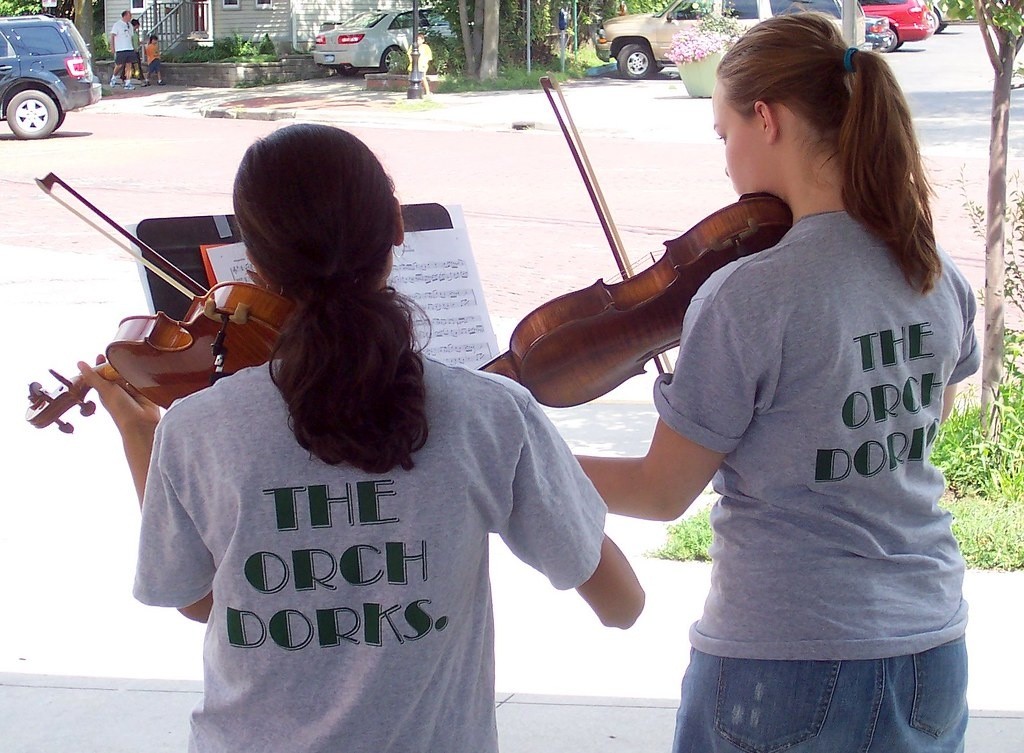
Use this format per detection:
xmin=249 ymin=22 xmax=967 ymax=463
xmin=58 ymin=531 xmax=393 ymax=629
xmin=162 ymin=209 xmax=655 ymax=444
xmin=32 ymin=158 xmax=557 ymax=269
xmin=419 ymin=13 xmax=430 ymax=27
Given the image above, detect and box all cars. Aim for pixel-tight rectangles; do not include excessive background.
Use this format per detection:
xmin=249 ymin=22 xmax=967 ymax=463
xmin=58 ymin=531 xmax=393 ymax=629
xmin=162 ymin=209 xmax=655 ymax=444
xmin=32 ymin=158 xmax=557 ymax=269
xmin=865 ymin=16 xmax=896 ymax=54
xmin=860 ymin=0 xmax=939 ymax=53
xmin=311 ymin=6 xmax=458 ymax=76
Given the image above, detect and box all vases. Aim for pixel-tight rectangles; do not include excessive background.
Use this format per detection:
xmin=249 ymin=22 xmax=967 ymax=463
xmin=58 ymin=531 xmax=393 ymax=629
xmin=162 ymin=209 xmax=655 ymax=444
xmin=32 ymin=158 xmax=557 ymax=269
xmin=672 ymin=51 xmax=729 ymax=99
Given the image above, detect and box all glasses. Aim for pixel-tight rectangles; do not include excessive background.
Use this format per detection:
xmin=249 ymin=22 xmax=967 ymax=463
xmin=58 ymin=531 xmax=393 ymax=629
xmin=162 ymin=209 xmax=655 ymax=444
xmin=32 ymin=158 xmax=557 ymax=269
xmin=127 ymin=22 xmax=129 ymax=29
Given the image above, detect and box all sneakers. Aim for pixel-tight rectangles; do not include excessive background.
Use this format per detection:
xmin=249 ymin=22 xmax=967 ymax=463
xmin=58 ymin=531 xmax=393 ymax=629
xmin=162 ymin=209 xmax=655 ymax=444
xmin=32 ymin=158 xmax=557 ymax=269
xmin=110 ymin=80 xmax=123 ymax=87
xmin=124 ymin=83 xmax=136 ymax=89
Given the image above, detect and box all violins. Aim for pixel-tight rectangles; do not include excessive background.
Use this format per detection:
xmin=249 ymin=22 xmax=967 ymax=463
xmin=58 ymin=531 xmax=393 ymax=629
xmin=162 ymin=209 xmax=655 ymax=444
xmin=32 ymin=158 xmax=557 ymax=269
xmin=467 ymin=188 xmax=792 ymax=411
xmin=19 ymin=277 xmax=305 ymax=439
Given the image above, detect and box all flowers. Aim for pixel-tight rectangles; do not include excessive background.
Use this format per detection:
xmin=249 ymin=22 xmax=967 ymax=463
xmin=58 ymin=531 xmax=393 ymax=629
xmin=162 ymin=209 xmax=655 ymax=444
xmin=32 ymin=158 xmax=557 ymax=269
xmin=663 ymin=1 xmax=743 ymax=64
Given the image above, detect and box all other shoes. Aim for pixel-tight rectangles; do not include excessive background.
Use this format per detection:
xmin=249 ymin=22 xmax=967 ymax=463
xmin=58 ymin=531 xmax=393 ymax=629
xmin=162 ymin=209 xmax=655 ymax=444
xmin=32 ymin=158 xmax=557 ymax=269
xmin=146 ymin=82 xmax=151 ymax=86
xmin=157 ymin=79 xmax=165 ymax=84
xmin=136 ymin=82 xmax=147 ymax=87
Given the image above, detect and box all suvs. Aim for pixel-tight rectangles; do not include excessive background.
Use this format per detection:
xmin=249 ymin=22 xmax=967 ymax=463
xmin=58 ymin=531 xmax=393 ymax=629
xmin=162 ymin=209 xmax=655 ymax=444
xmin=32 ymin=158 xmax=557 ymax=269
xmin=0 ymin=13 xmax=103 ymax=141
xmin=593 ymin=0 xmax=875 ymax=81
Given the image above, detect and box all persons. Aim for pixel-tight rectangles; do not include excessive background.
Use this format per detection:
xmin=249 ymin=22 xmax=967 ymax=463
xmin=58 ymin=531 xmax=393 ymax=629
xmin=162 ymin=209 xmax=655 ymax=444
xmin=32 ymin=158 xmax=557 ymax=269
xmin=76 ymin=124 xmax=647 ymax=752
xmin=144 ymin=35 xmax=166 ymax=86
xmin=573 ymin=14 xmax=982 ymax=752
xmin=110 ymin=11 xmax=148 ymax=90
xmin=407 ymin=33 xmax=433 ymax=98
